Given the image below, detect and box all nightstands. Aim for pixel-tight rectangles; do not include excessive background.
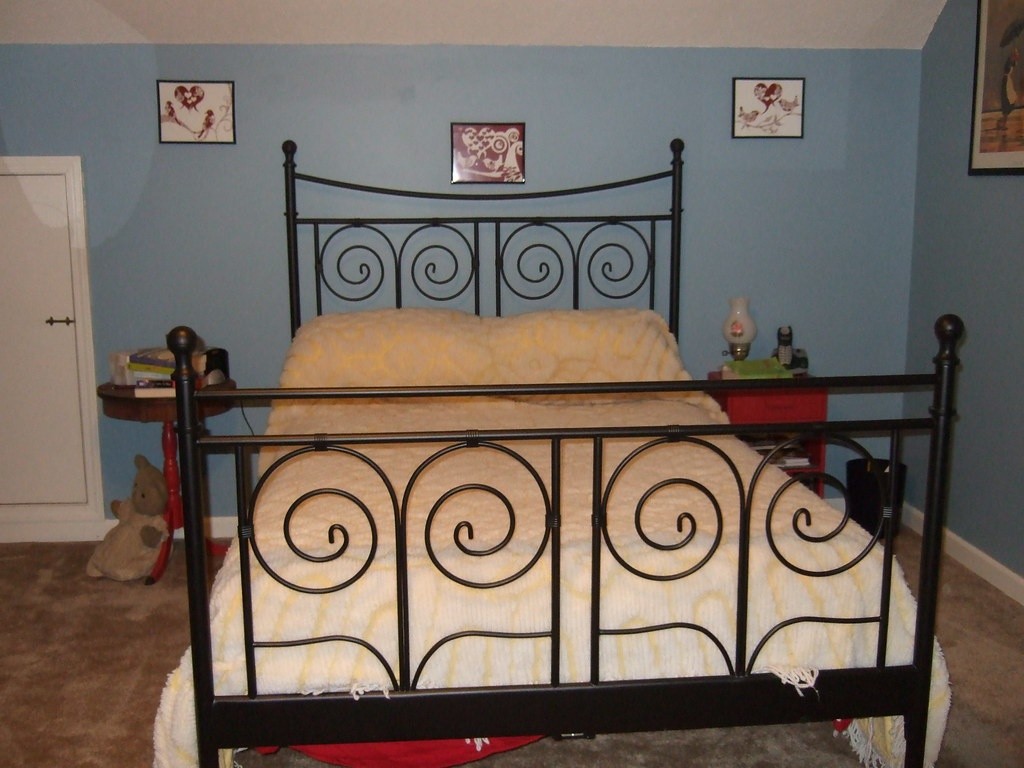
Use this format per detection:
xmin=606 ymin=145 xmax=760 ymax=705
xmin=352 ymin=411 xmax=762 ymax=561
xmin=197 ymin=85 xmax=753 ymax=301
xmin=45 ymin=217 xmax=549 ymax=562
xmin=710 ymin=370 xmax=827 ymax=493
xmin=96 ymin=384 xmax=234 ymax=585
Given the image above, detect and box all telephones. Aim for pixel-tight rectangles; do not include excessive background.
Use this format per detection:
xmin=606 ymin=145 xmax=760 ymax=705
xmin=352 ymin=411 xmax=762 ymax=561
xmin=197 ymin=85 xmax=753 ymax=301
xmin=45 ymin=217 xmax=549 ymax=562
xmin=771 ymin=325 xmax=809 ymax=374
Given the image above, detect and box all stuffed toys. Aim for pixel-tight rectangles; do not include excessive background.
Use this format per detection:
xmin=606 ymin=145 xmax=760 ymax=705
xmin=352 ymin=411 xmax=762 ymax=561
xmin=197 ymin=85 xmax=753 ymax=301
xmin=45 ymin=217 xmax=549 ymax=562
xmin=87 ymin=455 xmax=168 ymax=582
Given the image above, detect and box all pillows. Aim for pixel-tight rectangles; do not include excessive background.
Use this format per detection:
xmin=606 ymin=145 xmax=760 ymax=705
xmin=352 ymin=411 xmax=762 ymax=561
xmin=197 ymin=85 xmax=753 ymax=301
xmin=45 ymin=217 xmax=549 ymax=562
xmin=279 ymin=306 xmax=487 ymax=389
xmin=488 ymin=308 xmax=706 ymax=402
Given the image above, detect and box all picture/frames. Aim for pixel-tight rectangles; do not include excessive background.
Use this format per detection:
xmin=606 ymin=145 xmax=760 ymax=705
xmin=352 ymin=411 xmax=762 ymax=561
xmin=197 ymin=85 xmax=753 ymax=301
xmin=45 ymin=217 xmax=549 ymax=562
xmin=966 ymin=0 xmax=1024 ymax=177
xmin=731 ymin=77 xmax=806 ymax=138
xmin=155 ymin=78 xmax=237 ymax=143
xmin=449 ymin=120 xmax=527 ymax=186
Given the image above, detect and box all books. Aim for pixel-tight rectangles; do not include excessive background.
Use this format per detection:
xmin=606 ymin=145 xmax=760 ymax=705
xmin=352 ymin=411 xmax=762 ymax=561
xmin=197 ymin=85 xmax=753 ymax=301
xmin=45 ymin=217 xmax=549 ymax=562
xmin=126 ymin=347 xmax=207 ymax=398
xmin=722 ymin=357 xmax=792 ymax=379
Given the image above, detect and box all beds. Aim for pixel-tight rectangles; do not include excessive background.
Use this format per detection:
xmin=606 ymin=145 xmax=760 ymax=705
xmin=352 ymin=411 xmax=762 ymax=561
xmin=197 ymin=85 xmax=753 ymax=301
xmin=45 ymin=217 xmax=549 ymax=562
xmin=146 ymin=132 xmax=969 ymax=768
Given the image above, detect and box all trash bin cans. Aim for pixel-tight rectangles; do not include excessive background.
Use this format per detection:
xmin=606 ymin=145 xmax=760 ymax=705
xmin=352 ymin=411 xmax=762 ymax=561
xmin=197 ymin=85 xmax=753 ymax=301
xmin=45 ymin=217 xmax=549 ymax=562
xmin=847 ymin=458 xmax=910 ymax=539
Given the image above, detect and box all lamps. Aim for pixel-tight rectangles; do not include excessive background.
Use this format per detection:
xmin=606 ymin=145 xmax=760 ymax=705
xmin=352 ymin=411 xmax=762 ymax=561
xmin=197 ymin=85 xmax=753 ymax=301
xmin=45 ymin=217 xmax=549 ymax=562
xmin=721 ymin=297 xmax=756 ymax=361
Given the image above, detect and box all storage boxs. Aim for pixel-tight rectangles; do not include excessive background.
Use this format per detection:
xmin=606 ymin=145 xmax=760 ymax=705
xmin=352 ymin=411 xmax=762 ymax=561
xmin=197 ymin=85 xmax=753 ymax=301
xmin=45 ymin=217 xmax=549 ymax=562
xmin=109 ymin=351 xmax=137 ymax=386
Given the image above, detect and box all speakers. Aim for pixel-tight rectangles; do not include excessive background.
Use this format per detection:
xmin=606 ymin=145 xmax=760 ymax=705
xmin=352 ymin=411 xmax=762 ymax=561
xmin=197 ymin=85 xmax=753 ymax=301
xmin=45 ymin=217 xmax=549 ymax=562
xmin=204 ymin=349 xmax=229 ymax=386
